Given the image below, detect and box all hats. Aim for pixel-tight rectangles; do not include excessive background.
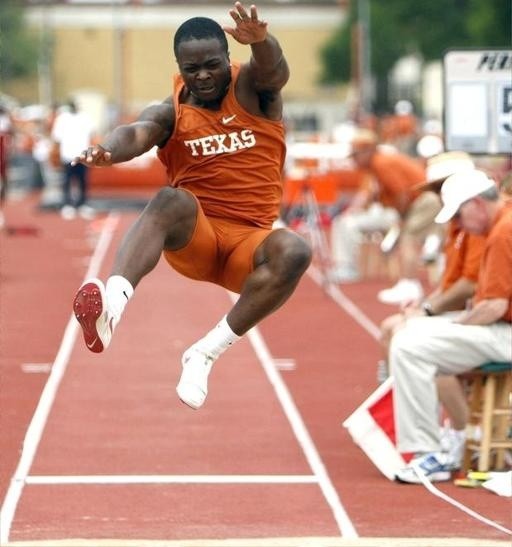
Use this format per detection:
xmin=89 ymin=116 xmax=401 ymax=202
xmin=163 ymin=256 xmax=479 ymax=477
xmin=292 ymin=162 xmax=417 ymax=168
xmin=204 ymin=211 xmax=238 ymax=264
xmin=434 ymin=168 xmax=496 ymax=224
xmin=347 ymin=141 xmax=378 ymax=158
xmin=411 ymin=149 xmax=473 ymax=192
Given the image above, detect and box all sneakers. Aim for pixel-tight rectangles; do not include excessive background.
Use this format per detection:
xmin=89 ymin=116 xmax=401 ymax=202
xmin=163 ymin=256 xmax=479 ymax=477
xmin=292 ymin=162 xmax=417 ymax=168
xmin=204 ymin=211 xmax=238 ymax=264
xmin=73 ymin=277 xmax=117 ymax=353
xmin=484 ymin=469 xmax=511 ymax=498
xmin=395 ymin=451 xmax=450 ymax=483
xmin=375 ymin=281 xmax=424 ymax=303
xmin=439 ymin=424 xmax=484 ymax=468
xmin=176 ymin=340 xmax=219 ymax=407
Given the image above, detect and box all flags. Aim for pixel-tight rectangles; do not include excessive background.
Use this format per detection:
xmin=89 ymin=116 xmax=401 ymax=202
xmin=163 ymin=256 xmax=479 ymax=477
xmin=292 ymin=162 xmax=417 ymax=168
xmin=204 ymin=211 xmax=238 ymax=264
xmin=341 ymin=372 xmax=452 ymax=484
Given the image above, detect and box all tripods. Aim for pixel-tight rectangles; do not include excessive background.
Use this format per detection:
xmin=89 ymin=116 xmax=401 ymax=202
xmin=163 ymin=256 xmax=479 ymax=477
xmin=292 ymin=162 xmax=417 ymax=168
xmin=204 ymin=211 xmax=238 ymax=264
xmin=283 ymin=184 xmax=334 ymax=293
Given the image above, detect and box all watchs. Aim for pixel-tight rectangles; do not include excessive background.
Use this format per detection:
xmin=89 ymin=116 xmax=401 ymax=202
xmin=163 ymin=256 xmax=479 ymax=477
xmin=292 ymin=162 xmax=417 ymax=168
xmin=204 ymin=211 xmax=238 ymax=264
xmin=423 ymin=303 xmax=433 ymax=317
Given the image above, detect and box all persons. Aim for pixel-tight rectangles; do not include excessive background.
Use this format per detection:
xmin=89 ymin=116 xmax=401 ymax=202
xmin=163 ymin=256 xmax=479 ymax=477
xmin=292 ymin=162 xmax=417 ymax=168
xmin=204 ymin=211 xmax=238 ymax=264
xmin=389 ymin=171 xmax=511 ymax=483
xmin=0 ymin=94 xmax=91 ymax=220
xmin=69 ymin=2 xmax=314 ymax=414
xmin=283 ymin=102 xmax=512 ymax=325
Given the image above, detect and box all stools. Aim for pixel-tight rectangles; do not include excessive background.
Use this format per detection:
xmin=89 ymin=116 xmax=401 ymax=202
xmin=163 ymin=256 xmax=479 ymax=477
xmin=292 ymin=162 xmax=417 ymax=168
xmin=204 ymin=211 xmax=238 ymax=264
xmin=359 ymin=229 xmax=392 ymax=285
xmin=452 ymin=363 xmax=512 ymax=476
